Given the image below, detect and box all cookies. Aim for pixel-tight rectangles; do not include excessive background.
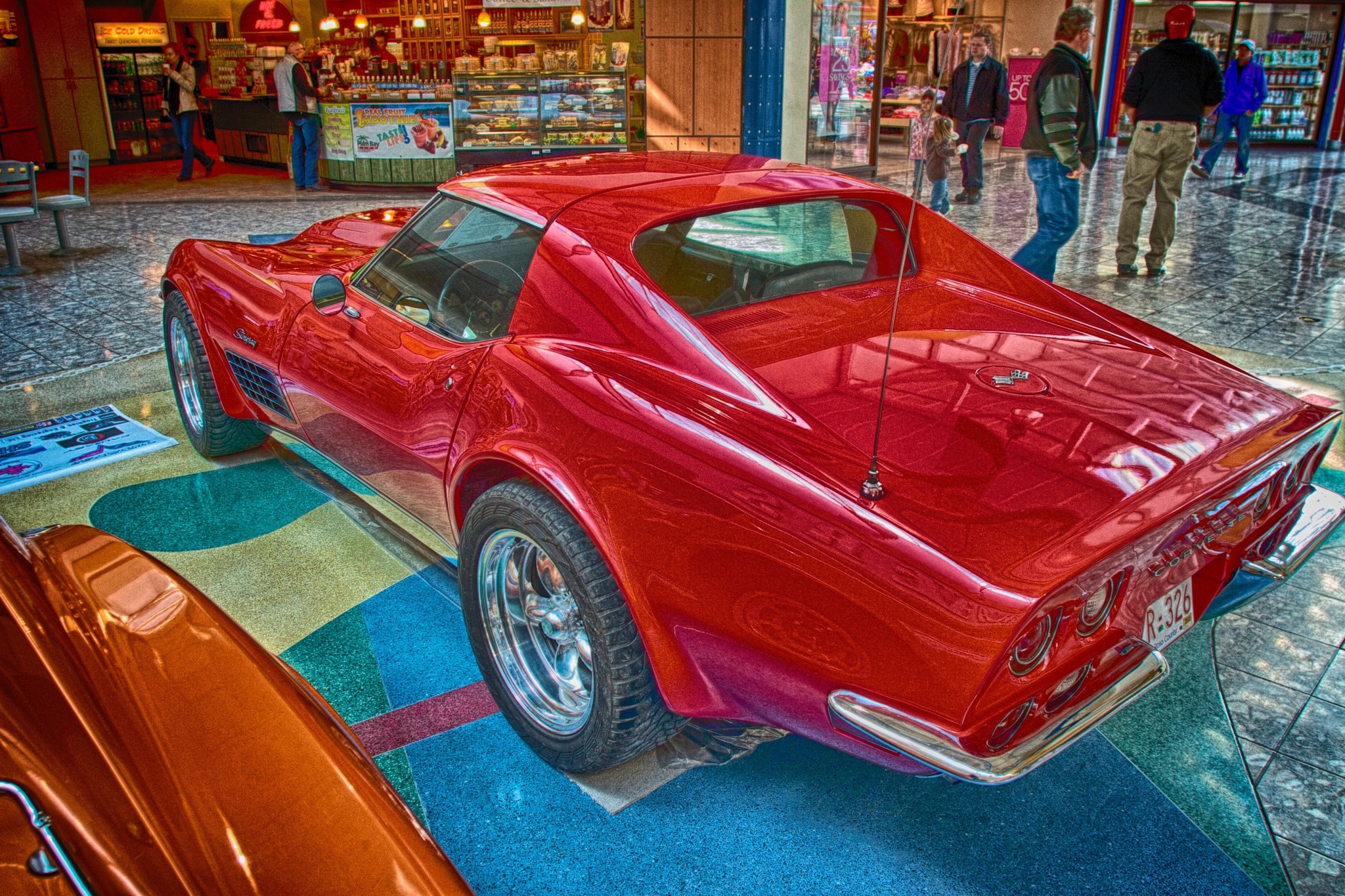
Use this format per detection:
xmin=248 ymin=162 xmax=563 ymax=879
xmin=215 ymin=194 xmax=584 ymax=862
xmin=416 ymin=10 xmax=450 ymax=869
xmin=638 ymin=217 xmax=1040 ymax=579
xmin=454 ymin=55 xmax=539 ymax=71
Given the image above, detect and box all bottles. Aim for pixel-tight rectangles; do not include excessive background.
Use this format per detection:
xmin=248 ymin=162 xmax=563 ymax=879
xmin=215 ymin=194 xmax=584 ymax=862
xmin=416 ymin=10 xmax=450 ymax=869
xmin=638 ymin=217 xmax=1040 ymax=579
xmin=208 ymin=38 xmax=247 ymax=57
xmin=470 ymin=13 xmax=507 ymax=35
xmin=1275 ymin=128 xmax=1304 ymax=140
xmin=1253 ymin=109 xmax=1305 ymax=126
xmin=102 ymin=54 xmax=165 ymax=109
xmin=513 ymin=9 xmax=552 ymax=34
xmin=353 ymin=75 xmax=453 ymax=98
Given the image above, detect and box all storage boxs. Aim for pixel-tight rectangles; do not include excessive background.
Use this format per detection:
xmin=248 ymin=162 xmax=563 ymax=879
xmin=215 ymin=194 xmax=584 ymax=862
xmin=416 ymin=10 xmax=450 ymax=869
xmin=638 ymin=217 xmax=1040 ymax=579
xmin=276 ymin=46 xmax=285 ymax=57
xmin=262 ymin=46 xmax=277 ymax=57
xmin=256 ymin=47 xmax=267 ymax=57
xmin=263 ymin=57 xmax=277 ymax=68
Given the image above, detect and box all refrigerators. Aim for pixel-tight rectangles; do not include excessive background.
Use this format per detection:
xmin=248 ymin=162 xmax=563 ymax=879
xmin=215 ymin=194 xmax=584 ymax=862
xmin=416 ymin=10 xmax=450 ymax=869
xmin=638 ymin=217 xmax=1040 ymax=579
xmin=88 ymin=19 xmax=184 ymax=165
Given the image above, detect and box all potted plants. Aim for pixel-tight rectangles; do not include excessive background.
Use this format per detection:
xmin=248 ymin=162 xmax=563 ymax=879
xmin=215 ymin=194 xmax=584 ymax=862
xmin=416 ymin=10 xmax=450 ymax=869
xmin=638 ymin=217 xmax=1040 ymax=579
xmin=631 ymin=50 xmax=637 ymax=62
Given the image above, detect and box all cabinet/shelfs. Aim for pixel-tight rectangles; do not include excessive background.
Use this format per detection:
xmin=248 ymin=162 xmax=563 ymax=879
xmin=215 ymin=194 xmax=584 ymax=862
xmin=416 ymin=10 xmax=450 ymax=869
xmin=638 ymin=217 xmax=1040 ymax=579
xmin=0 ymin=129 xmax=46 ymax=173
xmin=331 ymin=13 xmax=413 ymax=46
xmin=450 ymin=63 xmax=630 ymax=175
xmin=628 ymin=63 xmax=645 ymax=147
xmin=1310 ymin=40 xmax=1331 ymax=63
xmin=1203 ymin=64 xmax=1320 ymax=148
xmin=462 ymin=0 xmax=589 ymax=37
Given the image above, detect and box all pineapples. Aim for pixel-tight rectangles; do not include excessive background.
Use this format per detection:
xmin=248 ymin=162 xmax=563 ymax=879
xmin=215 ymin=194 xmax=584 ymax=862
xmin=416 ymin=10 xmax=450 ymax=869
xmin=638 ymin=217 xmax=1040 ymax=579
xmin=416 ymin=114 xmax=423 ymax=122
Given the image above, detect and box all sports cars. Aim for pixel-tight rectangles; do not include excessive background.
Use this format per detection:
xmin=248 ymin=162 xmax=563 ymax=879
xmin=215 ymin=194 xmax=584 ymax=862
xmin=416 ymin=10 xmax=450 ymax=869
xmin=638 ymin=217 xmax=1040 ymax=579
xmin=161 ymin=146 xmax=1344 ymax=789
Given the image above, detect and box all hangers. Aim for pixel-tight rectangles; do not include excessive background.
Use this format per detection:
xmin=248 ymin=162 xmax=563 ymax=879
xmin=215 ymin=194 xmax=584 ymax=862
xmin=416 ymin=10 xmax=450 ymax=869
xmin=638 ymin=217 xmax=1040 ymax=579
xmin=933 ymin=23 xmax=956 ymax=36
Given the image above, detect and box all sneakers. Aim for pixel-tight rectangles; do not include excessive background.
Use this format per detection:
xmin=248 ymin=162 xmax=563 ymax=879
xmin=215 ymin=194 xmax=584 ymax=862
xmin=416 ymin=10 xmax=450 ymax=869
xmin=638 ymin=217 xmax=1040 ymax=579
xmin=954 ymin=188 xmax=969 ymax=201
xmin=1148 ymin=265 xmax=1167 ymax=276
xmin=1117 ymin=263 xmax=1138 ymax=275
xmin=968 ymin=191 xmax=982 ymax=204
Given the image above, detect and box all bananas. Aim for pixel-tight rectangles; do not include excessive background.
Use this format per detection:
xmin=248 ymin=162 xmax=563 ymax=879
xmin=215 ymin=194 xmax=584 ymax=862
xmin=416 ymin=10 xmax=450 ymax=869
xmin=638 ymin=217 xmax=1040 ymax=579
xmin=436 ymin=128 xmax=445 ymax=148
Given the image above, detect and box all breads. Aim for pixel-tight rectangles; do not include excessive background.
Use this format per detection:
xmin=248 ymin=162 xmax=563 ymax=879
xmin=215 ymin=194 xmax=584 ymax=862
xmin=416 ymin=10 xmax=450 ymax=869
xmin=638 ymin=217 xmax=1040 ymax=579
xmin=544 ymin=96 xmax=625 ymax=108
xmin=551 ymin=116 xmax=578 ymax=127
xmin=599 ymin=121 xmax=614 ymax=126
xmin=464 ymin=84 xmax=538 ymax=143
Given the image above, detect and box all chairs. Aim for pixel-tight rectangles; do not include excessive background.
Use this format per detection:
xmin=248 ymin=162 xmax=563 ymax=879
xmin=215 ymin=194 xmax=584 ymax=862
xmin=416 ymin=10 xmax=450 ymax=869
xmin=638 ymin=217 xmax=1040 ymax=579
xmin=633 ymin=228 xmax=685 ymax=296
xmin=0 ymin=159 xmax=36 ymax=276
xmin=37 ymin=148 xmax=90 ymax=258
xmin=752 ymin=259 xmax=855 ymax=302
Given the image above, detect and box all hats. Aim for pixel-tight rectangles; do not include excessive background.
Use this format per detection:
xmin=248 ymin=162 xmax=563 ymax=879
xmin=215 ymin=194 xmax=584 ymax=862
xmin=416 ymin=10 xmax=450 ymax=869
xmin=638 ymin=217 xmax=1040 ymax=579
xmin=1241 ymin=39 xmax=1257 ymax=53
xmin=1164 ymin=3 xmax=1196 ymax=40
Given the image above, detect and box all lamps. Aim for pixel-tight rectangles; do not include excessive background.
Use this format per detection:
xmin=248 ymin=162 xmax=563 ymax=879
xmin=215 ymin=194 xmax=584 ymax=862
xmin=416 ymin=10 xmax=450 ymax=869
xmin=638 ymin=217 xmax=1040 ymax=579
xmin=318 ymin=19 xmax=330 ymax=31
xmin=412 ymin=10 xmax=427 ymax=28
xmin=289 ymin=0 xmax=302 ymax=33
xmin=354 ymin=0 xmax=369 ymax=29
xmin=324 ymin=1 xmax=340 ymax=30
xmin=477 ymin=7 xmax=491 ymax=28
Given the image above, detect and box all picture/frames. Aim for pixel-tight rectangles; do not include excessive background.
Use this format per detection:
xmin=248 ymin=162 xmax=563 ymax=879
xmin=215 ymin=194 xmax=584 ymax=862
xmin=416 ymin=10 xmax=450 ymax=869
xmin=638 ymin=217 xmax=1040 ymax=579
xmin=419 ymin=28 xmax=427 ymax=39
xmin=435 ymin=40 xmax=445 ymax=61
xmin=425 ymin=17 xmax=434 ymax=39
xmin=399 ymin=18 xmax=410 ymax=41
xmin=398 ymin=0 xmax=461 ymax=18
xmin=445 ymin=39 xmax=454 ymax=61
xmin=452 ymin=15 xmax=461 ymax=39
xmin=558 ymin=10 xmax=583 ymax=34
xmin=636 ymin=45 xmax=645 ymax=62
xmin=433 ymin=17 xmax=442 ymax=39
xmin=401 ymin=40 xmax=411 ymax=61
xmin=453 ymin=39 xmax=462 ymax=58
xmin=409 ymin=40 xmax=420 ymax=61
xmin=639 ymin=17 xmax=645 ymax=42
xmin=408 ymin=18 xmax=419 ymax=39
xmin=419 ymin=40 xmax=428 ymax=61
xmin=427 ymin=39 xmax=436 ymax=61
xmin=442 ymin=16 xmax=453 ymax=39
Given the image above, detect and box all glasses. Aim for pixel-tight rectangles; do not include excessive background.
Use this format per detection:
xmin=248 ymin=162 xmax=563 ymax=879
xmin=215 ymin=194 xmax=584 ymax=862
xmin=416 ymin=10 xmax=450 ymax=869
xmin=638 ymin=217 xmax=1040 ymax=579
xmin=1082 ymin=27 xmax=1096 ymax=40
xmin=297 ymin=47 xmax=305 ymax=51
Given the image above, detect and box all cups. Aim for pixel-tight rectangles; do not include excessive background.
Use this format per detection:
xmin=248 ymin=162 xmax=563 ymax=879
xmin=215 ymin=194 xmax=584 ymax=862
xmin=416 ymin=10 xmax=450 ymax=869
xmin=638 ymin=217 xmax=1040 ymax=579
xmin=163 ymin=63 xmax=170 ymax=75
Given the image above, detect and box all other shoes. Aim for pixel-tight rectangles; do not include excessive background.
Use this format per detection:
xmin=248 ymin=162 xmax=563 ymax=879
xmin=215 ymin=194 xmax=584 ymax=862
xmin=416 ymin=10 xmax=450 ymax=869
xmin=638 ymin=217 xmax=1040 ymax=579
xmin=204 ymin=159 xmax=215 ymax=177
xmin=1191 ymin=164 xmax=1210 ymax=178
xmin=295 ymin=184 xmax=306 ymax=191
xmin=306 ymin=183 xmax=330 ymax=191
xmin=939 ymin=213 xmax=944 ymax=217
xmin=177 ymin=174 xmax=190 ymax=181
xmin=1232 ymin=172 xmax=1245 ymax=179
xmin=945 ymin=204 xmax=954 ymax=216
xmin=910 ymin=191 xmax=921 ymax=199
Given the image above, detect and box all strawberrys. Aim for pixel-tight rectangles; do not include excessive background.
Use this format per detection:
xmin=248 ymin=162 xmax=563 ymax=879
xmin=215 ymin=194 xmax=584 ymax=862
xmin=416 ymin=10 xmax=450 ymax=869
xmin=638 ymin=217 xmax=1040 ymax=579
xmin=424 ymin=142 xmax=435 ymax=154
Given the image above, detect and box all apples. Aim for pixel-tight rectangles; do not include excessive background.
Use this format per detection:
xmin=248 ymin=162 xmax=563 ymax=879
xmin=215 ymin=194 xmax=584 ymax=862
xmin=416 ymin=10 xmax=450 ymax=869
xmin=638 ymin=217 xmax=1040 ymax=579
xmin=568 ymin=82 xmax=590 ymax=90
xmin=574 ymin=138 xmax=626 ymax=145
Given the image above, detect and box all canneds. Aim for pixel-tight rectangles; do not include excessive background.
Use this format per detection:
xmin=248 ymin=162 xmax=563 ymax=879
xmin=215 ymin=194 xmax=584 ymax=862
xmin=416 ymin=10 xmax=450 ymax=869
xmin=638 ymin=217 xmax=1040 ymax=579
xmin=546 ymin=80 xmax=567 ymax=91
xmin=117 ymin=119 xmax=144 ymax=131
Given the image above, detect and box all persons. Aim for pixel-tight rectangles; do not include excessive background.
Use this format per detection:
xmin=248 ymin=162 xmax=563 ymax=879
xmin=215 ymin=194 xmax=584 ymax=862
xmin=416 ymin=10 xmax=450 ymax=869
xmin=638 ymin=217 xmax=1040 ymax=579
xmin=1190 ymin=38 xmax=1268 ymax=180
xmin=910 ymin=33 xmax=1010 ymax=219
xmin=160 ymin=41 xmax=216 ymax=181
xmin=813 ymin=3 xmax=854 ymax=153
xmin=359 ymin=30 xmax=396 ymax=77
xmin=1009 ymin=6 xmax=1101 ymax=284
xmin=273 ymin=42 xmax=333 ymax=192
xmin=1114 ymin=4 xmax=1223 ymax=276
